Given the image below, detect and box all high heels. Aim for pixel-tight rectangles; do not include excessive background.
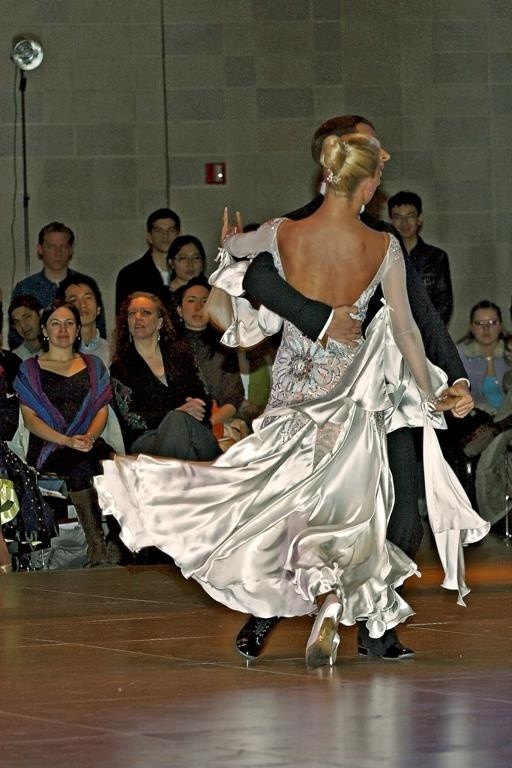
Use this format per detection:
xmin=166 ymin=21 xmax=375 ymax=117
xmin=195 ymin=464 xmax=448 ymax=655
xmin=305 ymin=594 xmax=344 ymax=669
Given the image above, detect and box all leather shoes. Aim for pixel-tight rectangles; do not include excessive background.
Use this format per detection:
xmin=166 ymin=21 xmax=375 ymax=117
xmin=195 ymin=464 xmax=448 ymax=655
xmin=358 ymin=620 xmax=416 ymax=661
xmin=236 ymin=615 xmax=285 ymax=660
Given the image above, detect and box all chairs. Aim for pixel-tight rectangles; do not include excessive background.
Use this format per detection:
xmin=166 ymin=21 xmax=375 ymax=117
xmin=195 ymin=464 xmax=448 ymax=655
xmin=38 ymin=472 xmax=73 ymax=537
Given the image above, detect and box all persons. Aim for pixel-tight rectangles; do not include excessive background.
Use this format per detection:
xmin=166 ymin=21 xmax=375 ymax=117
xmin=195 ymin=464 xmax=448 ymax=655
xmin=94 ymin=113 xmax=512 ymax=669
xmin=1 ymin=208 xmax=282 ymax=576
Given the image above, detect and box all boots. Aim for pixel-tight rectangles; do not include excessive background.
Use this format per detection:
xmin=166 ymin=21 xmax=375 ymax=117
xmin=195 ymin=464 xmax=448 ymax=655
xmin=70 ymin=487 xmax=111 ymax=568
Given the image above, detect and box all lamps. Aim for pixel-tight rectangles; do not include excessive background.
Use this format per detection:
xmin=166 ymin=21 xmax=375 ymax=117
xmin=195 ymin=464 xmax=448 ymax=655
xmin=10 ymin=32 xmax=44 ymax=277
